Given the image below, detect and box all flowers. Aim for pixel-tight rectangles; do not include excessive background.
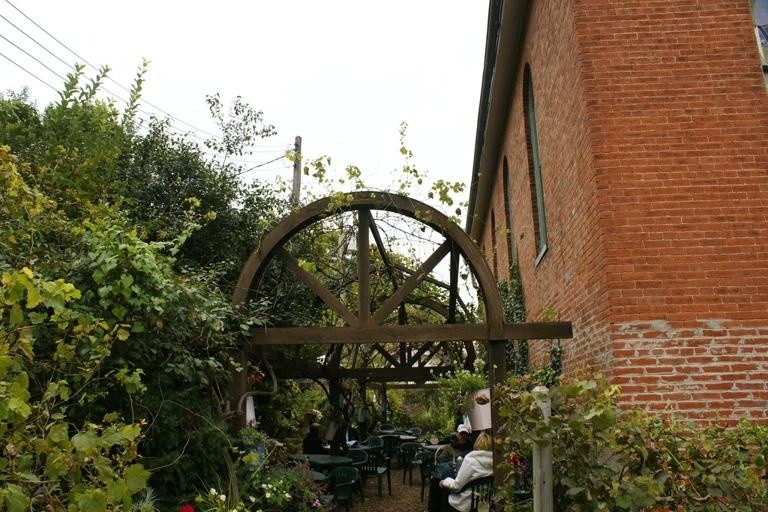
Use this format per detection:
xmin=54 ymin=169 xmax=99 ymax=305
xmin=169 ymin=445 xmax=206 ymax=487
xmin=425 ymin=357 xmax=492 ymax=417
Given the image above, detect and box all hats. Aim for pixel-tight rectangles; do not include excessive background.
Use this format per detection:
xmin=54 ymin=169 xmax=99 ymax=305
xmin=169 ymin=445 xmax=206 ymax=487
xmin=456 ymin=423 xmax=470 ymax=435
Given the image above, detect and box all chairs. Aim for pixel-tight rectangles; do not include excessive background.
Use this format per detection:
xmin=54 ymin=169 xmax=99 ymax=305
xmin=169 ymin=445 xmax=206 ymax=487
xmin=284 ymin=425 xmax=462 ymax=512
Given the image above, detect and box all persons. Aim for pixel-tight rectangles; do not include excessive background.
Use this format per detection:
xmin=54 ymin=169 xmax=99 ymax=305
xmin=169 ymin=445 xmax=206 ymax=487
xmin=428 ymin=424 xmax=493 ymax=511
xmin=333 ymin=419 xmax=360 ymax=448
xmin=303 ymin=422 xmax=338 ymax=455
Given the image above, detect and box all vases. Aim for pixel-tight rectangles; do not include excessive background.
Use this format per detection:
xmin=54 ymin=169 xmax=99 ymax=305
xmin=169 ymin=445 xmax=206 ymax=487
xmin=464 ymin=388 xmax=492 ymax=432
